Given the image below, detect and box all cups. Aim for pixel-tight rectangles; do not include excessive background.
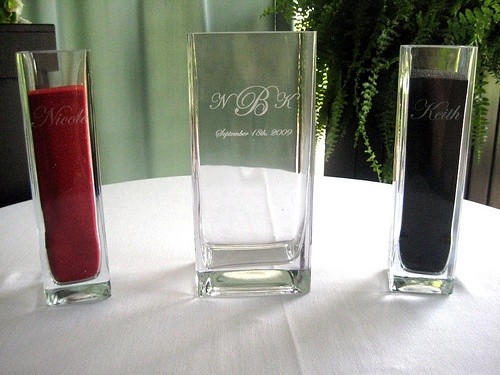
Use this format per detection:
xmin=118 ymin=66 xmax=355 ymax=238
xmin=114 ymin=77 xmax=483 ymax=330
xmin=187 ymin=31 xmax=313 ymax=298
xmin=15 ymin=48 xmax=112 ymax=309
xmin=386 ymin=44 xmax=480 ymax=294
